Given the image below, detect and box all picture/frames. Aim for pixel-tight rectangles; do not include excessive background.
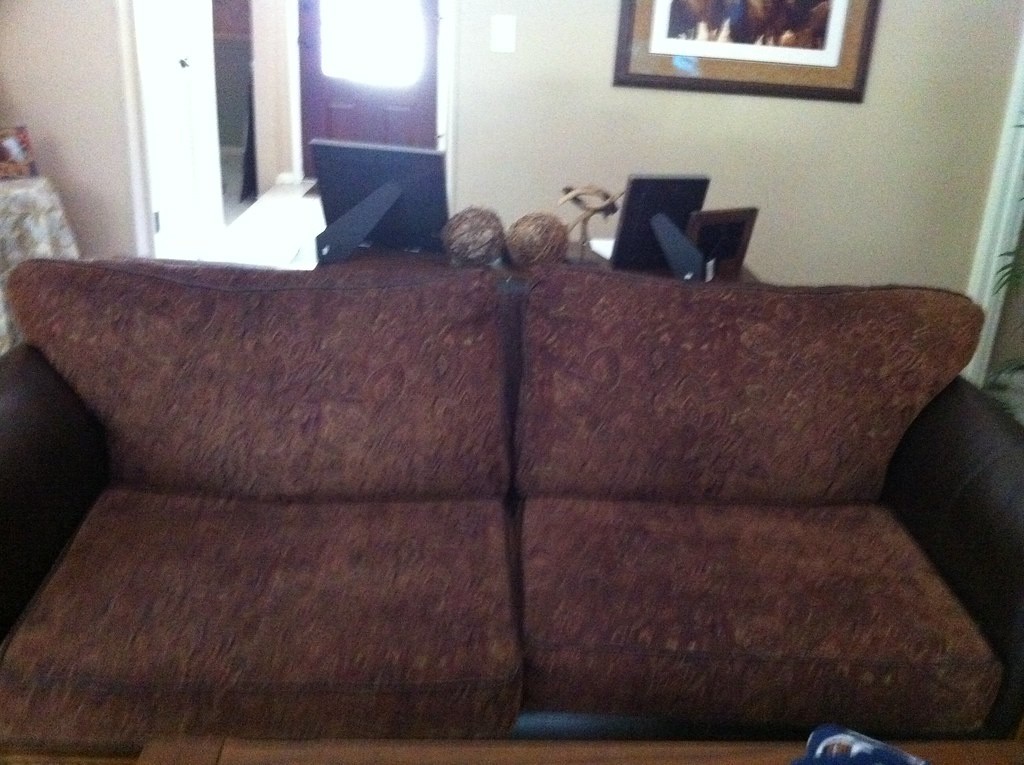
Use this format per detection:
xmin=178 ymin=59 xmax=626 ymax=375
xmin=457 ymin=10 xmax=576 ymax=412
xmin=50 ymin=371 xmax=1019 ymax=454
xmin=677 ymin=207 xmax=757 ymax=281
xmin=307 ymin=137 xmax=450 ymax=262
xmin=610 ymin=175 xmax=710 ymax=278
xmin=612 ymin=0 xmax=879 ymax=104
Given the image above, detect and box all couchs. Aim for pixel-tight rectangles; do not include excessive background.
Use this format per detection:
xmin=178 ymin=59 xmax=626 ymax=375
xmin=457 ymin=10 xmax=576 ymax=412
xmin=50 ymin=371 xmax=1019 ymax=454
xmin=0 ymin=252 xmax=1024 ymax=755
